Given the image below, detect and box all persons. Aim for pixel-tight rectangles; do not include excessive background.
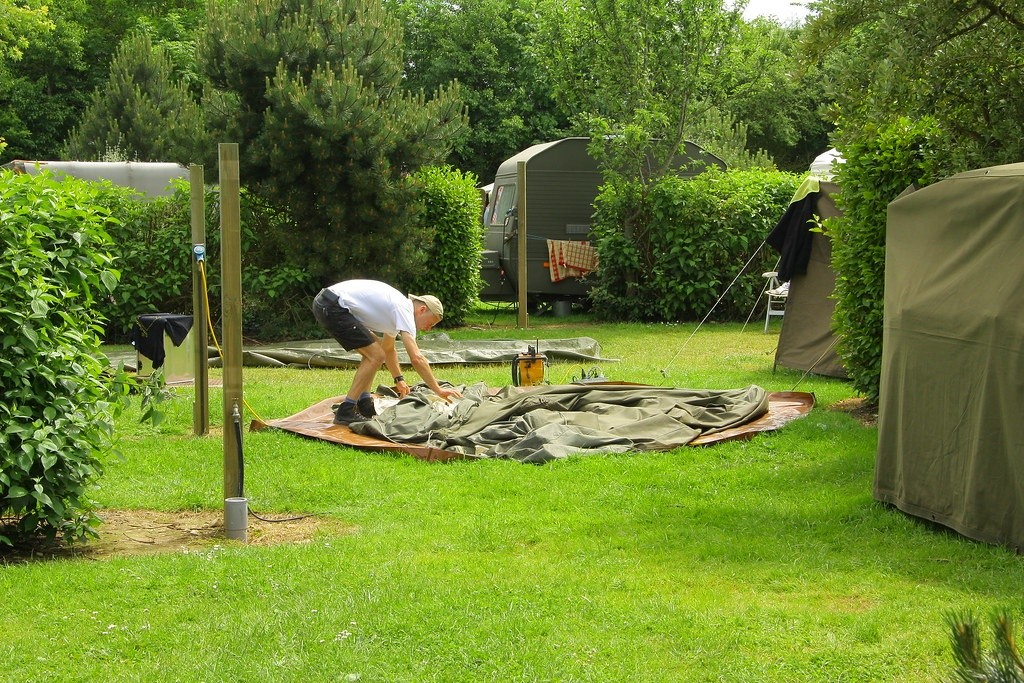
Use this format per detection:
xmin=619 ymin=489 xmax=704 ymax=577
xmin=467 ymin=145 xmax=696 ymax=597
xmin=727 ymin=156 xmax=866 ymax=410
xmin=312 ymin=279 xmax=463 ymax=425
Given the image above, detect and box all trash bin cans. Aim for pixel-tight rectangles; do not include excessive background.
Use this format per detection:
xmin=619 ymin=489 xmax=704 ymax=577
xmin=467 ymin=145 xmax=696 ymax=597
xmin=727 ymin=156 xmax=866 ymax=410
xmin=129 ymin=312 xmax=196 ymax=385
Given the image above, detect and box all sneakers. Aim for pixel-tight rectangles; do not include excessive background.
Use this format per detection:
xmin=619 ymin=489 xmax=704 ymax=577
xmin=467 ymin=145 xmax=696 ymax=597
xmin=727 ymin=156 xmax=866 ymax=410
xmin=333 ymin=397 xmax=379 ymax=426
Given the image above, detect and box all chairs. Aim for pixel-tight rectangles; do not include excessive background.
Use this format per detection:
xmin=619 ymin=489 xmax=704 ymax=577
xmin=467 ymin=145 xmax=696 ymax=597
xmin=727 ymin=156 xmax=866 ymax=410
xmin=762 ymin=271 xmax=790 ymax=331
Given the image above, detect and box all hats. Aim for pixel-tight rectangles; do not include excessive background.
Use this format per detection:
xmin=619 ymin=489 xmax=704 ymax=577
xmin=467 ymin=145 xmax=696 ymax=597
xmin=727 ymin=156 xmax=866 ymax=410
xmin=408 ymin=294 xmax=443 ymax=321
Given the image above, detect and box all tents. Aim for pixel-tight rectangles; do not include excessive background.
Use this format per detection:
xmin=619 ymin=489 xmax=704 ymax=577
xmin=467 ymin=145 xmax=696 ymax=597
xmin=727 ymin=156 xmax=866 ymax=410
xmin=661 ymin=147 xmax=855 ymax=382
xmin=872 ymin=161 xmax=1024 ymax=554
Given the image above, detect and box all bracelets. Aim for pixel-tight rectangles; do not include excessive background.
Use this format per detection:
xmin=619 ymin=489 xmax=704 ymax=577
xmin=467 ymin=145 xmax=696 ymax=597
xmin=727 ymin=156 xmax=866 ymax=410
xmin=393 ymin=376 xmax=404 ymax=383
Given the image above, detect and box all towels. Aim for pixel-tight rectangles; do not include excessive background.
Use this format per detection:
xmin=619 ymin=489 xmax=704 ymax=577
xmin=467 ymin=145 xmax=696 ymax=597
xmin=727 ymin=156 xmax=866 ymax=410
xmin=546 ymin=238 xmax=599 ymax=283
